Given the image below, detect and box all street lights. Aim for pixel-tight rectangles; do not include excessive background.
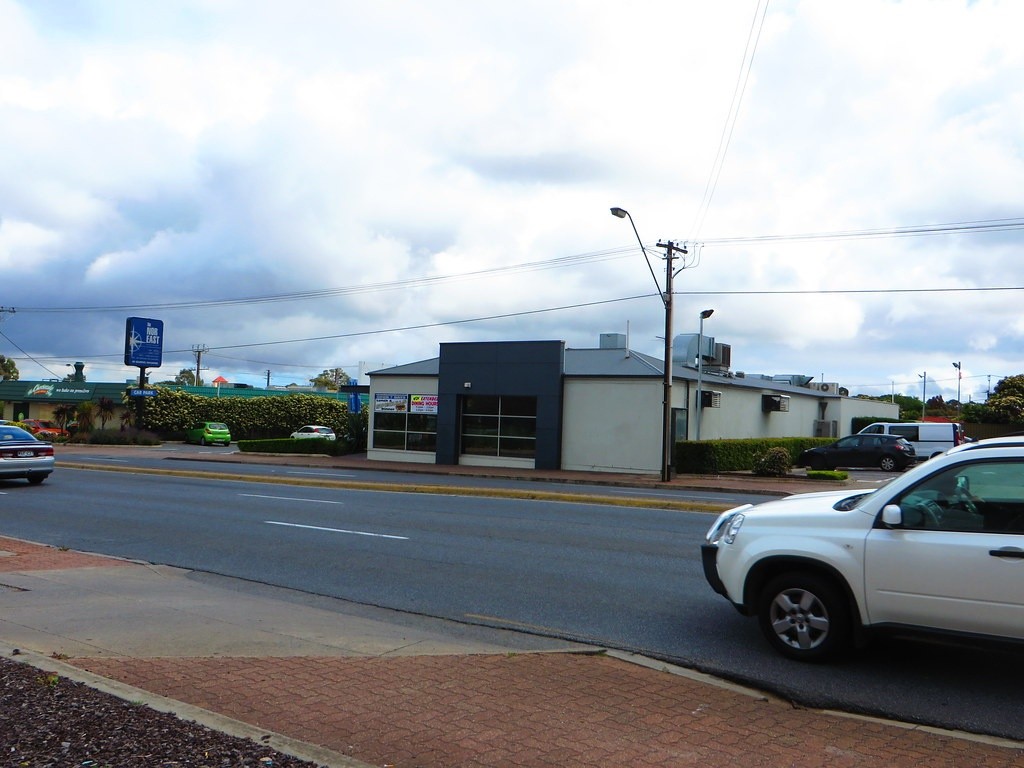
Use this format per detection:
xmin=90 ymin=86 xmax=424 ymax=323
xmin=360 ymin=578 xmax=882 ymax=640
xmin=952 ymin=361 xmax=961 ymax=421
xmin=608 ymin=206 xmax=674 ymax=483
xmin=919 ymin=371 xmax=926 ymax=423
xmin=695 ymin=308 xmax=715 ymax=441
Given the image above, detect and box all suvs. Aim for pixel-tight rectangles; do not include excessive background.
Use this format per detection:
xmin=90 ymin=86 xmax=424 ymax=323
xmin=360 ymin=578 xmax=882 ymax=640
xmin=799 ymin=433 xmax=917 ymax=471
xmin=700 ymin=435 xmax=1024 ymax=665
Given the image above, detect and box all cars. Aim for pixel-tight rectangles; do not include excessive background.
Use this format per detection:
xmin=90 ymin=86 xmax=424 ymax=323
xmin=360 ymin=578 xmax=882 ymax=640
xmin=183 ymin=421 xmax=231 ymax=447
xmin=289 ymin=423 xmax=336 ymax=441
xmin=0 ymin=424 xmax=55 ymax=484
xmin=20 ymin=418 xmax=70 ymax=437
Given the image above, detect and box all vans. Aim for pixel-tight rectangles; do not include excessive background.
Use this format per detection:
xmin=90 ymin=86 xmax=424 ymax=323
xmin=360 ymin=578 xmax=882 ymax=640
xmin=838 ymin=422 xmax=967 ymax=462
